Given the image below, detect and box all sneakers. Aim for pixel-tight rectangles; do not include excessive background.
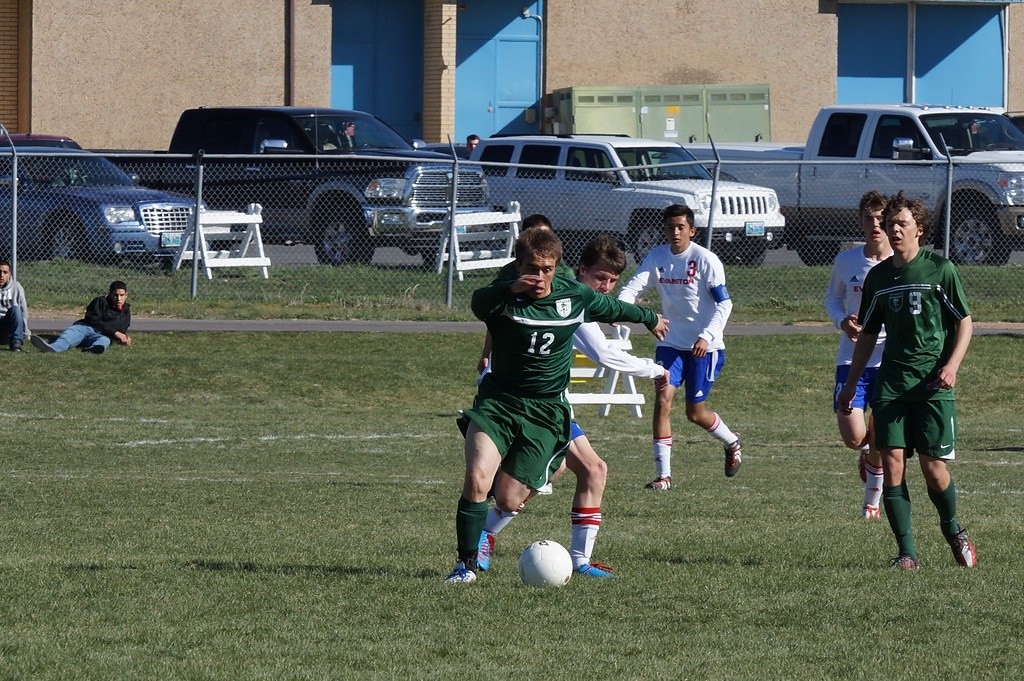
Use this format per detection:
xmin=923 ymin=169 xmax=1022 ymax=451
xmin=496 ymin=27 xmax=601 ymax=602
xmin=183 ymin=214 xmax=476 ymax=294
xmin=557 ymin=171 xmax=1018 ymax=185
xmin=82 ymin=345 xmax=105 ymax=354
xmin=726 ymin=432 xmax=742 ymax=477
xmin=31 ymin=336 xmax=55 ymax=352
xmin=574 ymin=561 xmax=616 ymax=578
xmin=941 ymin=527 xmax=975 ymax=568
xmin=859 ymin=451 xmax=867 ymax=482
xmin=444 ymin=561 xmax=476 ymax=583
xmin=478 ymin=529 xmax=496 ymax=571
xmin=863 ymin=504 xmax=880 ymax=519
xmin=645 ymin=476 xmax=671 ymax=490
xmin=889 ymin=555 xmax=919 ymax=569
xmin=538 ymin=483 xmax=553 ymax=494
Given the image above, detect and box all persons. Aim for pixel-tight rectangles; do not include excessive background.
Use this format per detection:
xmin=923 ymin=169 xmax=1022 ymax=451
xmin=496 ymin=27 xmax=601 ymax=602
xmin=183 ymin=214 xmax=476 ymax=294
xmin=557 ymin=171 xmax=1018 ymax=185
xmin=840 ymin=189 xmax=976 ymax=571
xmin=825 ymin=190 xmax=894 ymax=522
xmin=472 ymin=237 xmax=625 ymax=572
xmin=467 ymin=134 xmax=479 ymax=160
xmin=477 ymin=214 xmax=567 ymax=495
xmin=0 ymin=261 xmax=31 ymax=351
xmin=608 ymin=203 xmax=742 ymax=490
xmin=30 ymin=280 xmax=132 ymax=354
xmin=443 ymin=229 xmax=671 ymax=585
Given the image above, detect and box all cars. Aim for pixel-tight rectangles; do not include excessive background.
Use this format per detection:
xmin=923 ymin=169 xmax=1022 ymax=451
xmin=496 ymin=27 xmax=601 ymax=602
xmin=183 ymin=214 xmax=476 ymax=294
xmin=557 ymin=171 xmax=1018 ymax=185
xmin=0 ymin=133 xmax=83 ymax=149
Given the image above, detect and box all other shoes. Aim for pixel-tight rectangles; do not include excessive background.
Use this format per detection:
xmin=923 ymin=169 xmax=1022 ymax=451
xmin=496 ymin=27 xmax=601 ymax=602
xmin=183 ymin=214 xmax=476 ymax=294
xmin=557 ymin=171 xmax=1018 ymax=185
xmin=9 ymin=340 xmax=20 ymax=352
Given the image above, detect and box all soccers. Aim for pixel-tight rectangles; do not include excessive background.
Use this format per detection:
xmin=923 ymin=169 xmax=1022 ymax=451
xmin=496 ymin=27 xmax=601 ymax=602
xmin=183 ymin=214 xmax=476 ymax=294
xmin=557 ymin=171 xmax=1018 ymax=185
xmin=517 ymin=539 xmax=574 ymax=588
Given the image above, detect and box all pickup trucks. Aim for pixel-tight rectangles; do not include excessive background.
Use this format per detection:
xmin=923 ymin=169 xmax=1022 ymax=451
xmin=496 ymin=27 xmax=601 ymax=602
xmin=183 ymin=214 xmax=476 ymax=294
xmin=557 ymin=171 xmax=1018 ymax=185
xmin=681 ymin=103 xmax=1024 ymax=266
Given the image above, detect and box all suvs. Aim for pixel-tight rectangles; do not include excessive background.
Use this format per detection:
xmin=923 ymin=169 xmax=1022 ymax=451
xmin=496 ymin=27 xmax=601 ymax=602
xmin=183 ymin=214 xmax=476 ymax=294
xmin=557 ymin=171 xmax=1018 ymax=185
xmin=469 ymin=133 xmax=786 ymax=266
xmin=167 ymin=105 xmax=493 ymax=267
xmin=0 ymin=146 xmax=214 ymax=269
